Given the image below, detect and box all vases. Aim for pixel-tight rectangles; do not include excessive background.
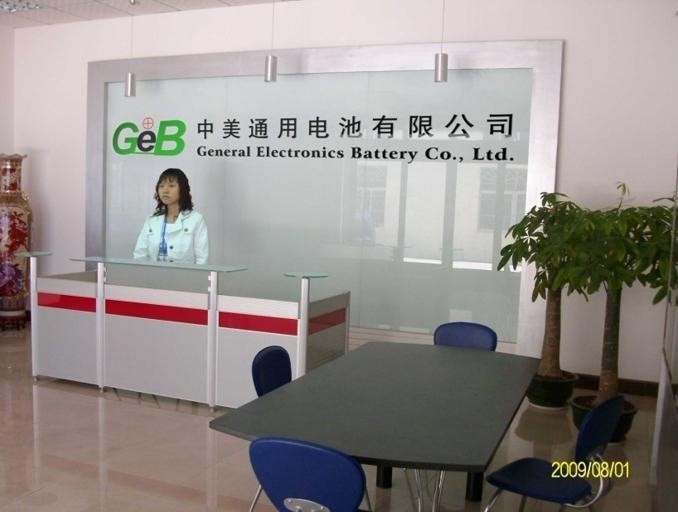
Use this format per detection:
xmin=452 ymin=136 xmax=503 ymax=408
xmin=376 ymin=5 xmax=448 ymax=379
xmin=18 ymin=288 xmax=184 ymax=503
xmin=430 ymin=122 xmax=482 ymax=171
xmin=0 ymin=152 xmax=34 ymax=322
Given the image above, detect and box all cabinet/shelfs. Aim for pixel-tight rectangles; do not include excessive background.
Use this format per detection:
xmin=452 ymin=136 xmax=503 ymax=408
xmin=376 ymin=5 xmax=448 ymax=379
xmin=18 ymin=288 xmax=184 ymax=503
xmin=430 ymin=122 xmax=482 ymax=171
xmin=644 ymin=194 xmax=677 ymax=511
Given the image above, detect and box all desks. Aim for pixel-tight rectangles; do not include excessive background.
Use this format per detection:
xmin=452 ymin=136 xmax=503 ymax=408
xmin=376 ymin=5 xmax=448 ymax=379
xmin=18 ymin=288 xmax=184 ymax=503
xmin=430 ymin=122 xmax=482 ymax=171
xmin=206 ymin=340 xmax=540 ymax=512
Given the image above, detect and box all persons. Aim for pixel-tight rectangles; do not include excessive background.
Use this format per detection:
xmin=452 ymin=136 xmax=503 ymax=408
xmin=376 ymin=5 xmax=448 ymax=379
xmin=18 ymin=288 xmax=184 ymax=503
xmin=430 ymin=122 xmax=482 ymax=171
xmin=132 ymin=168 xmax=210 ymax=265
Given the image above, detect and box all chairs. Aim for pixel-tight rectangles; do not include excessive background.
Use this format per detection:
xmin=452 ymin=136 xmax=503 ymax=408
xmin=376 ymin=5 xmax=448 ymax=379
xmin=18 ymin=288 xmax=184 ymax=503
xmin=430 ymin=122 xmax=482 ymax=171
xmin=481 ymin=395 xmax=638 ymax=512
xmin=248 ymin=346 xmax=371 ymax=512
xmin=247 ymin=437 xmax=371 ymax=512
xmin=431 ymin=321 xmax=498 ymax=351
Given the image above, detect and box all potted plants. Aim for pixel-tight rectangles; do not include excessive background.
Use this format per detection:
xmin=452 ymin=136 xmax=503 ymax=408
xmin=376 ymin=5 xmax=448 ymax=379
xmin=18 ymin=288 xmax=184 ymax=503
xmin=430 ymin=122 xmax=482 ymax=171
xmin=560 ymin=179 xmax=678 ymax=439
xmin=494 ymin=190 xmax=596 ymax=409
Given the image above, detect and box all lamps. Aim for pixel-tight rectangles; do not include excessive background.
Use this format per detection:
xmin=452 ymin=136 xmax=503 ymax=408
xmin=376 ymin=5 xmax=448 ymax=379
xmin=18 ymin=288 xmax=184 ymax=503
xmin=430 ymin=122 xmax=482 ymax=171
xmin=123 ymin=0 xmax=139 ymax=98
xmin=432 ymin=1 xmax=448 ymax=84
xmin=264 ymin=0 xmax=277 ymax=82
